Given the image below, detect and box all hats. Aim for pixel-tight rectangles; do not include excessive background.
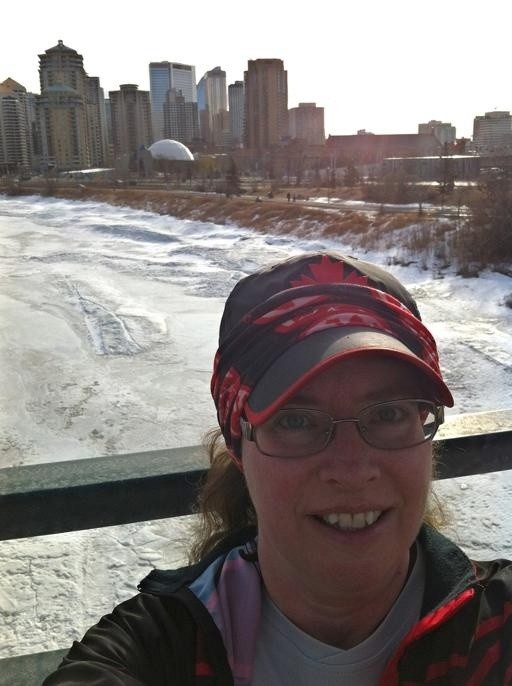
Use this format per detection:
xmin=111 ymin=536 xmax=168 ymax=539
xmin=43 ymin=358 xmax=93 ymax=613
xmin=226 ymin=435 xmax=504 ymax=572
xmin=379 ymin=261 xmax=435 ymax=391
xmin=209 ymin=253 xmax=454 ymax=449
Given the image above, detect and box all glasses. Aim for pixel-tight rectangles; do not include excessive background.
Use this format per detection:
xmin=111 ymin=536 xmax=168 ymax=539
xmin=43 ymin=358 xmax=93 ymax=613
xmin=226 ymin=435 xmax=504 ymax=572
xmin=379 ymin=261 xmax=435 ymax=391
xmin=239 ymin=399 xmax=443 ymax=459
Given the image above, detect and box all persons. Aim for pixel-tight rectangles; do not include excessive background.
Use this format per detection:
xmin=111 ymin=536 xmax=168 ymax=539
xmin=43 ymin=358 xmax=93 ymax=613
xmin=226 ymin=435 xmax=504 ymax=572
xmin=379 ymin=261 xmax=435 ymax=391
xmin=38 ymin=249 xmax=512 ymax=685
